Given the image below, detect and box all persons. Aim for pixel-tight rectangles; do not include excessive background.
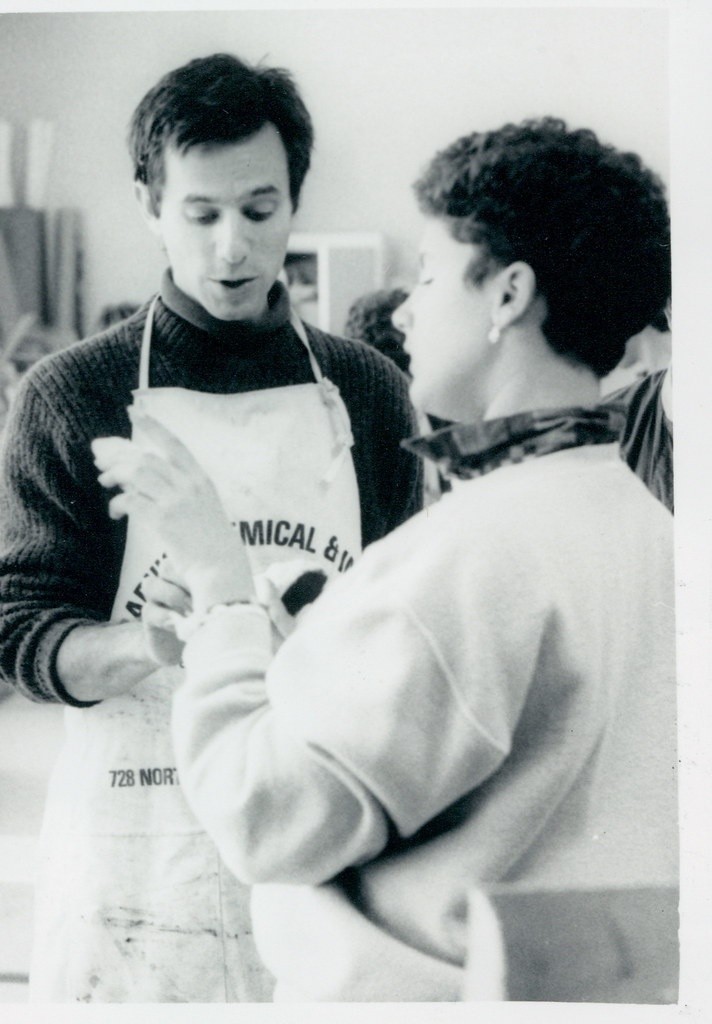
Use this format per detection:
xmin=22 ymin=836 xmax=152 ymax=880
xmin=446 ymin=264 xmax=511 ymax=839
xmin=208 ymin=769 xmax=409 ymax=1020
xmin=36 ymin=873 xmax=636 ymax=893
xmin=87 ymin=121 xmax=680 ymax=1002
xmin=345 ymin=290 xmax=409 ymax=371
xmin=2 ymin=54 xmax=424 ymax=1003
